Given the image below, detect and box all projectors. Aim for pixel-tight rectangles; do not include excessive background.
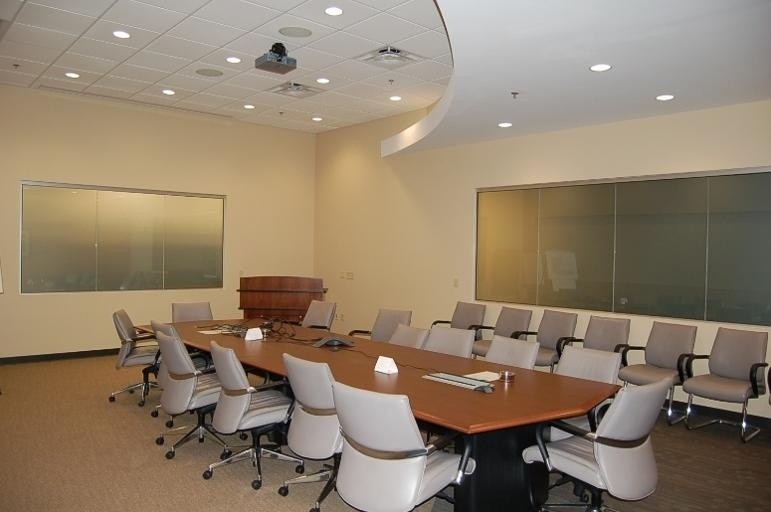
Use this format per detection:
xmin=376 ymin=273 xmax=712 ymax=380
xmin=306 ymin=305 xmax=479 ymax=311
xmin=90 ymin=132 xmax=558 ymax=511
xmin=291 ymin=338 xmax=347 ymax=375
xmin=255 ymin=53 xmax=296 ymax=74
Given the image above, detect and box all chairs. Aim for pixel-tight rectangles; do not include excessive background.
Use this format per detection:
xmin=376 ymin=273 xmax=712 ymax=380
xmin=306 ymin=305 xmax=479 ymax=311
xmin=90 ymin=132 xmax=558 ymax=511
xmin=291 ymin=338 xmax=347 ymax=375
xmin=483 ymin=334 xmax=541 ymax=371
xmin=618 ymin=319 xmax=698 ymax=427
xmin=277 ymin=351 xmax=342 ymax=510
xmin=110 ymin=308 xmax=162 ymax=408
xmin=348 ymin=302 xmax=413 ymax=347
xmin=420 ymin=324 xmax=477 ymax=359
xmin=296 ymin=301 xmax=337 ymax=331
xmin=562 ymin=314 xmax=632 ymax=388
xmin=387 ymin=322 xmax=430 ymax=350
xmin=550 ymin=345 xmax=623 ymax=441
xmin=430 ymin=301 xmax=486 ymax=360
xmin=200 ymin=340 xmax=294 ymax=492
xmin=149 ymin=318 xmax=209 ymax=429
xmin=156 ymin=330 xmax=249 ymax=461
xmin=474 ymin=305 xmax=532 ymax=358
xmin=332 ymin=378 xmax=474 ymax=511
xmin=684 ymin=326 xmax=770 ymax=444
xmin=521 ymin=378 xmax=673 ymax=509
xmin=510 ymin=309 xmax=578 ymax=375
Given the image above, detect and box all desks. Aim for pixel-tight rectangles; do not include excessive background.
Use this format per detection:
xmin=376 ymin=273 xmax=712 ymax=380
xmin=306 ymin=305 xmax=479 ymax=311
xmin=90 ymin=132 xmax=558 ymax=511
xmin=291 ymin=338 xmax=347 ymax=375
xmin=131 ymin=318 xmax=621 ymax=507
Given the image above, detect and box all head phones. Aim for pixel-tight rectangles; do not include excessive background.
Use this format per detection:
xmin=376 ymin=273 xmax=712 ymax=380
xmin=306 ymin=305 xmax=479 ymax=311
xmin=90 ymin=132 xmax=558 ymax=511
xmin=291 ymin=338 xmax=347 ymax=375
xmin=261 ymin=317 xmax=283 ymax=338
